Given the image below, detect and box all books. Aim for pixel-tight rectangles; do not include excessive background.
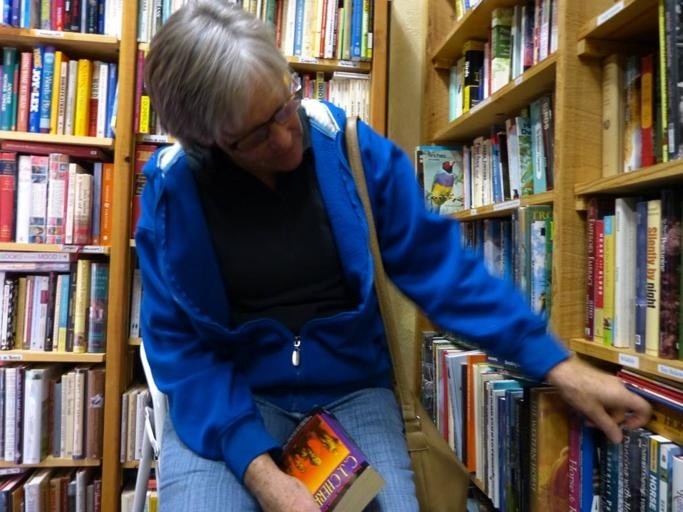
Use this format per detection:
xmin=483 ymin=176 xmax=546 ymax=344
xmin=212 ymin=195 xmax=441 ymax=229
xmin=128 ymin=269 xmax=146 ymax=336
xmin=272 ymin=405 xmax=385 ymax=512
xmin=136 ymin=1 xmax=374 ymax=136
xmin=133 ymin=145 xmax=158 ymax=240
xmin=120 ymin=384 xmax=158 ymax=512
xmin=0 ymin=363 xmax=105 ymax=511
xmin=2 ymin=1 xmax=121 ymax=138
xmin=0 ymin=139 xmax=113 ymax=247
xmin=413 ymin=1 xmax=683 ymax=511
xmin=0 ymin=251 xmax=109 ymax=353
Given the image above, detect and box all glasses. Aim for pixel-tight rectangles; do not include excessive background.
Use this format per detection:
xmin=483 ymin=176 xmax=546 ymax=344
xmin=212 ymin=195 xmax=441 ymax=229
xmin=228 ymin=70 xmax=304 ymax=152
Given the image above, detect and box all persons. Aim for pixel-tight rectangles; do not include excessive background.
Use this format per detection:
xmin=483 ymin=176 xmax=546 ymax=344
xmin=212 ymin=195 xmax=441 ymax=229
xmin=135 ymin=0 xmax=652 ymax=512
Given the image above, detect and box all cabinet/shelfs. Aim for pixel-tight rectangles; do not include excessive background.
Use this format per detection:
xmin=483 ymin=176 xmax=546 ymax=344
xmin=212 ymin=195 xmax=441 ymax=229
xmin=414 ymin=1 xmax=683 ymax=511
xmin=0 ymin=0 xmax=390 ymax=512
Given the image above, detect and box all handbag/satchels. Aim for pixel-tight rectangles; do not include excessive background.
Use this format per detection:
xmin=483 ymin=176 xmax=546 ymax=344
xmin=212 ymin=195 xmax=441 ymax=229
xmin=398 ymin=363 xmax=471 ymax=512
